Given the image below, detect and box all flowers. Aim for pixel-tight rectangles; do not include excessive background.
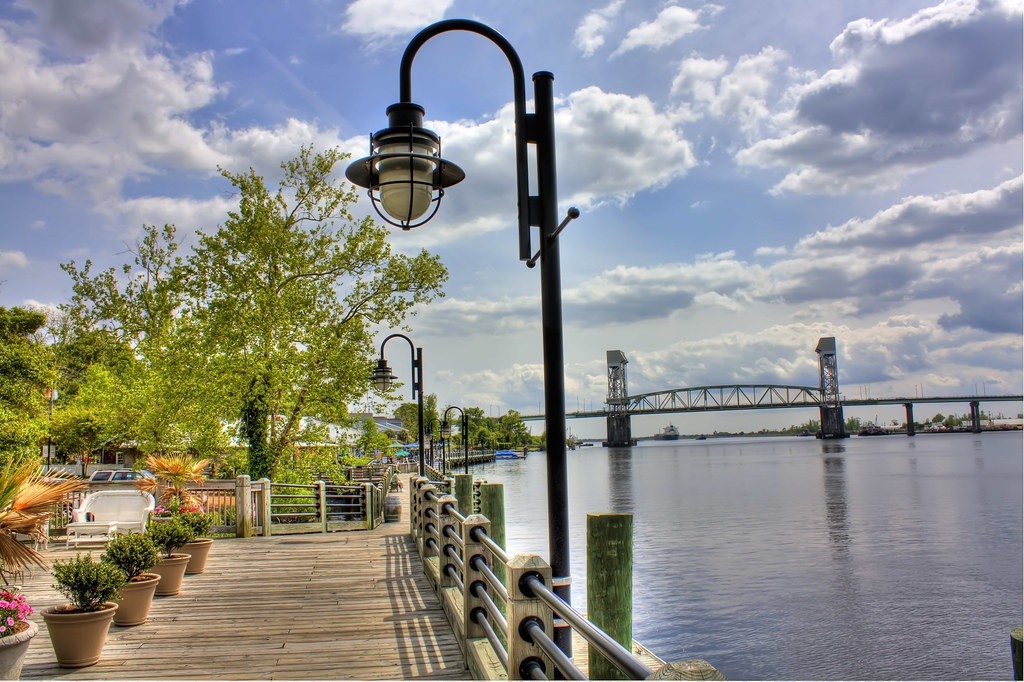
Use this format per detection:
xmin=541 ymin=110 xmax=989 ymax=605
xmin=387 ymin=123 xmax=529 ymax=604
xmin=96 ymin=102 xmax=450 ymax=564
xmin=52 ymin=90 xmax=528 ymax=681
xmin=152 ymin=505 xmax=204 ymax=518
xmin=0 ymin=584 xmax=37 ymax=639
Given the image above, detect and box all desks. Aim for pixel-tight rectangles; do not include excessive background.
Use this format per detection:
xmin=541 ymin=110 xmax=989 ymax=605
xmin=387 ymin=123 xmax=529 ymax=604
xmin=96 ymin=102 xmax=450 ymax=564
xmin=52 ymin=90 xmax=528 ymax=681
xmin=67 ymin=520 xmax=119 ymax=549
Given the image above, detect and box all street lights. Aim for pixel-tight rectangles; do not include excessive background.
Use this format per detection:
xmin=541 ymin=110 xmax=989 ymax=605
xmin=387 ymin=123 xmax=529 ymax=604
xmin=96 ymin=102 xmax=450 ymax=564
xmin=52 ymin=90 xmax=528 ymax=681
xmin=440 ymin=406 xmax=468 ymax=474
xmin=344 ymin=18 xmax=580 ymax=681
xmin=362 ymin=333 xmax=426 ymax=478
xmin=45 ymin=386 xmax=58 ymax=472
xmin=425 ymin=417 xmax=446 ymax=475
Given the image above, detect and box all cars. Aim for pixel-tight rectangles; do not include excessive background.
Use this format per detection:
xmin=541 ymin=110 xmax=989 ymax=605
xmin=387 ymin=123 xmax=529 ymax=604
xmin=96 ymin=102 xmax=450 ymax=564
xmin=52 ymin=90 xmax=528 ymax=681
xmin=87 ymin=468 xmax=155 ymax=481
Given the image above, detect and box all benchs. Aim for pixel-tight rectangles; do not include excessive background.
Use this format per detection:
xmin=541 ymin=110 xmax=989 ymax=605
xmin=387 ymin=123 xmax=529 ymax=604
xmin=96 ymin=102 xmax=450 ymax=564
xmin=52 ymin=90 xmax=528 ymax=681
xmin=73 ymin=490 xmax=156 ymax=545
xmin=3 ymin=500 xmax=50 ymax=551
xmin=390 ymin=482 xmax=398 ymax=492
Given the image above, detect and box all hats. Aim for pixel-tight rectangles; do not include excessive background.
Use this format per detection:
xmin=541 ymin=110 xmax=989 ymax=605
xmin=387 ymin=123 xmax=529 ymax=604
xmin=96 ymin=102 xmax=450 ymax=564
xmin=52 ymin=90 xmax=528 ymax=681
xmin=396 ymin=470 xmax=401 ymax=473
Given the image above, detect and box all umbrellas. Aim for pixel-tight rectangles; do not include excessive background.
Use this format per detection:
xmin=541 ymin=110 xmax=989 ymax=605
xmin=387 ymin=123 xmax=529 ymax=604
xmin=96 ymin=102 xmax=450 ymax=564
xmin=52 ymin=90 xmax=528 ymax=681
xmin=384 ymin=441 xmax=452 ymax=457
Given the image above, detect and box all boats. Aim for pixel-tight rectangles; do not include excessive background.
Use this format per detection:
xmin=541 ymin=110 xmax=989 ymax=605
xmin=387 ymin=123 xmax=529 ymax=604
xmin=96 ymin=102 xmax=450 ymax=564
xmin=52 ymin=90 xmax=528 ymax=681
xmin=495 ymin=451 xmax=520 ymax=459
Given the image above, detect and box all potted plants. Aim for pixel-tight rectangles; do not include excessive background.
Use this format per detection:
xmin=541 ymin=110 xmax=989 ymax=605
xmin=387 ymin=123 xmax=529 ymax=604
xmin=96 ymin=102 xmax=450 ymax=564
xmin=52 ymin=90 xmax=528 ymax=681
xmin=144 ymin=513 xmax=195 ymax=596
xmin=133 ymin=451 xmax=210 ymax=524
xmin=172 ymin=508 xmax=218 ymax=574
xmin=100 ymin=529 xmax=162 ymax=627
xmin=39 ymin=549 xmax=132 ymax=669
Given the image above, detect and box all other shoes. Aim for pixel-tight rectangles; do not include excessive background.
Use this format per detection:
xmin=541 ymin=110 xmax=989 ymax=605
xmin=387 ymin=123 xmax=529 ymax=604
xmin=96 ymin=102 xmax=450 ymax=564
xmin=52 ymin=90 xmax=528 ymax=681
xmin=400 ymin=488 xmax=405 ymax=492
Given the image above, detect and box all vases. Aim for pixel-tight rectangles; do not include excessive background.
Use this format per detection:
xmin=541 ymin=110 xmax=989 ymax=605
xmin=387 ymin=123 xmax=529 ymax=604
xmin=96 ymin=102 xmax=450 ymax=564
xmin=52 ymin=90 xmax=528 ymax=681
xmin=0 ymin=619 xmax=41 ymax=682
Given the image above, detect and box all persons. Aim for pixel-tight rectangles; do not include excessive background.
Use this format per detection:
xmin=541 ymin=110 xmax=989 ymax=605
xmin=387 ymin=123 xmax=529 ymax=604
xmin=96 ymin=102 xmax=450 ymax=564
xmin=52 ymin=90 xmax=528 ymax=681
xmin=389 ymin=470 xmax=403 ymax=494
xmin=396 ymin=457 xmax=411 ymax=473
